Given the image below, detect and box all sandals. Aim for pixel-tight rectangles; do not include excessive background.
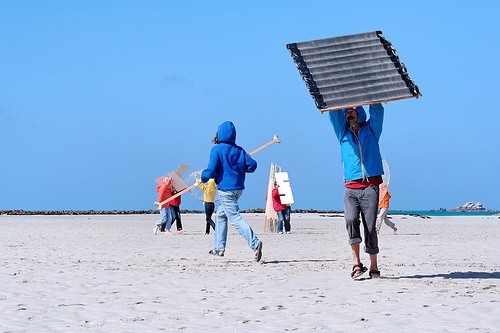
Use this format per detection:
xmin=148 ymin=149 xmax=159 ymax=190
xmin=369 ymin=270 xmax=380 ymax=279
xmin=351 ymin=263 xmax=368 ymax=279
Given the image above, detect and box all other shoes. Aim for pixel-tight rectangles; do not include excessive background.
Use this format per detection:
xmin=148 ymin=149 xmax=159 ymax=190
xmin=287 ymin=231 xmax=291 ymax=234
xmin=153 ymin=227 xmax=158 ymax=235
xmin=254 ymin=241 xmax=262 ymax=262
xmin=279 ymin=232 xmax=283 ymax=234
xmin=209 ymin=249 xmax=224 ymax=256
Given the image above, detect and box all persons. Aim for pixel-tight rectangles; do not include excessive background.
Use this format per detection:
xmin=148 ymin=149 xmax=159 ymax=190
xmin=196 ymin=176 xmax=218 ymax=235
xmin=200 ymin=121 xmax=262 ymax=263
xmin=273 ymin=181 xmax=291 ymax=235
xmin=153 ymin=176 xmax=186 ymax=237
xmin=328 ymin=102 xmax=385 ymax=280
xmin=376 ymin=181 xmax=397 ymax=235
